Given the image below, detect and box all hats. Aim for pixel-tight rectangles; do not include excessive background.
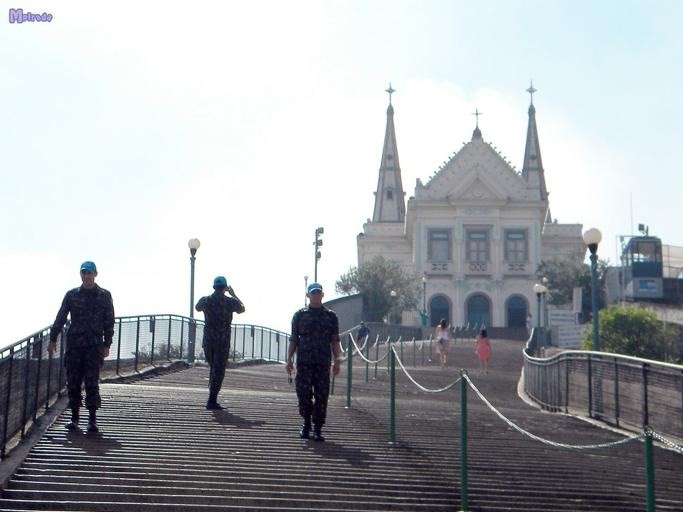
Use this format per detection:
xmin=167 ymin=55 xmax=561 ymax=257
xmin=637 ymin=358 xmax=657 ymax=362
xmin=308 ymin=283 xmax=322 ymax=293
xmin=81 ymin=261 xmax=96 ymax=272
xmin=214 ymin=276 xmax=226 ymax=286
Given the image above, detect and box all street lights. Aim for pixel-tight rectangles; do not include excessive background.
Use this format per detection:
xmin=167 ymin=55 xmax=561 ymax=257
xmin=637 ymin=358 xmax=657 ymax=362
xmin=313 ymin=227 xmax=323 ymax=282
xmin=187 ymin=238 xmax=199 ymax=363
xmin=582 ymin=227 xmax=601 ymax=351
xmin=533 ymin=276 xmax=548 ymax=358
xmin=303 ymin=275 xmax=308 ymax=308
xmin=390 ymin=290 xmax=396 ymax=342
xmin=421 ymin=276 xmax=427 ymax=339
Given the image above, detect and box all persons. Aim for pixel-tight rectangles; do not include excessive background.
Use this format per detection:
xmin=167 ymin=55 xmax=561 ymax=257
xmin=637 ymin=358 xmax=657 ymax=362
xmin=436 ymin=319 xmax=451 ymax=367
xmin=358 ymin=321 xmax=369 ymax=348
xmin=475 ymin=328 xmax=491 ymax=375
xmin=195 ymin=276 xmax=245 ymax=409
xmin=286 ymin=282 xmax=340 ymax=441
xmin=47 ymin=261 xmax=115 ymax=430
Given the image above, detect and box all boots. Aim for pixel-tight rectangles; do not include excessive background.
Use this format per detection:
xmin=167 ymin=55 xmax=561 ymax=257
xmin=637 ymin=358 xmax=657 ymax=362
xmin=300 ymin=417 xmax=310 ymax=437
xmin=313 ymin=425 xmax=324 ymax=441
xmin=206 ymin=391 xmax=219 ymax=408
xmin=87 ymin=408 xmax=98 ymax=431
xmin=66 ymin=408 xmax=79 ymax=429
